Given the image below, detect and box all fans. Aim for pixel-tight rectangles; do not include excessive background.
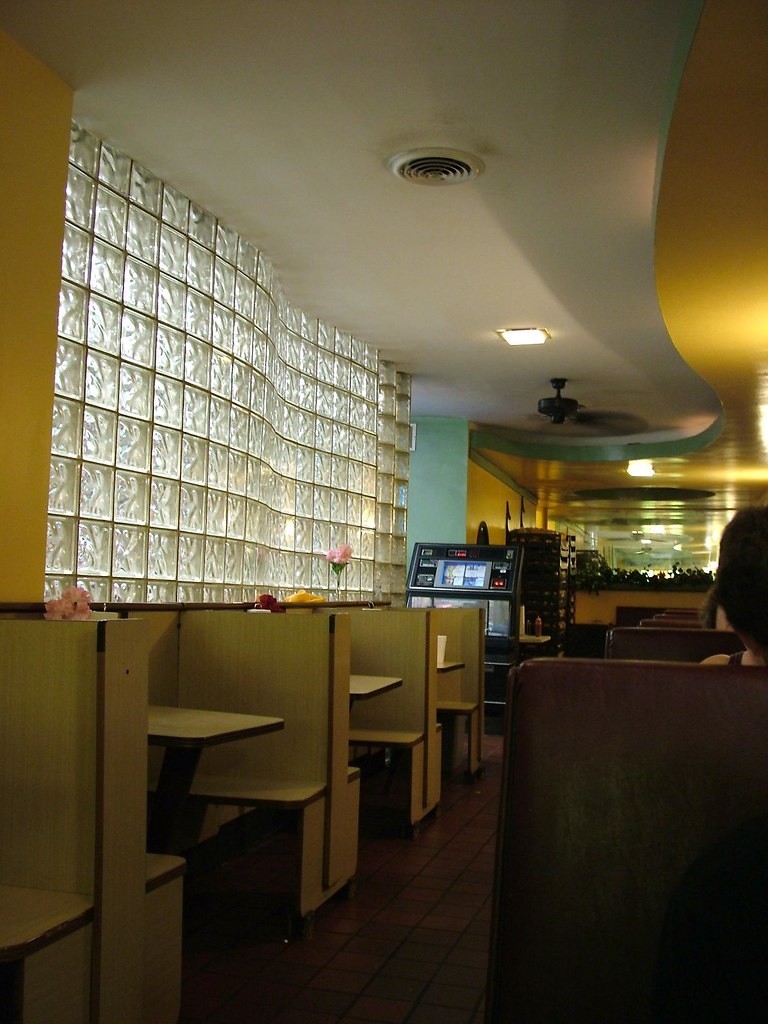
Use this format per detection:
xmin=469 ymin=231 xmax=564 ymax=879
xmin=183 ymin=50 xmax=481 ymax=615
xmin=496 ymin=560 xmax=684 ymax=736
xmin=475 ymin=377 xmax=650 ymax=441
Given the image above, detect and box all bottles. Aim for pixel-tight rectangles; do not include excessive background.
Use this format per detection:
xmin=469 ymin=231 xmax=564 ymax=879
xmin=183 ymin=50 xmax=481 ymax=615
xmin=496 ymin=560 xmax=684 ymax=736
xmin=535 ymin=616 xmax=542 ymax=636
xmin=525 ymin=620 xmax=530 ymax=634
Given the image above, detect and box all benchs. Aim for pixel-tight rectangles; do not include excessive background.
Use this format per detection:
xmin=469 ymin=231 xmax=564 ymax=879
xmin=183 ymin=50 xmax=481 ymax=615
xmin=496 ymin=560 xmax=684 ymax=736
xmin=493 ymin=607 xmax=768 ymax=1024
xmin=0 ymin=703 xmax=479 ymax=1024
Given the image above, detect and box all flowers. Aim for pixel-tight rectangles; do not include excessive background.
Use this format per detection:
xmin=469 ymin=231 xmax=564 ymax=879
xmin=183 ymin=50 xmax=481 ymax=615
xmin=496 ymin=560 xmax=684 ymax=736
xmin=42 ymin=587 xmax=95 ymax=622
xmin=254 ymin=594 xmax=285 ymax=614
xmin=325 ymin=543 xmax=354 ymax=599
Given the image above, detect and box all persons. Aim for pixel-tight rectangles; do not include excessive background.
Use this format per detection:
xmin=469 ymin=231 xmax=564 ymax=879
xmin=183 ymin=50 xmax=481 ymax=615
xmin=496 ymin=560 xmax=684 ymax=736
xmin=702 ymin=505 xmax=768 ymax=666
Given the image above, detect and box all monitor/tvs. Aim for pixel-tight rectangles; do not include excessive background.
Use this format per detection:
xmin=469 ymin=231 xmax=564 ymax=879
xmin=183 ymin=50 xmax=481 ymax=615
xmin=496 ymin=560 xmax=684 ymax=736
xmin=433 ymin=560 xmax=492 ymax=589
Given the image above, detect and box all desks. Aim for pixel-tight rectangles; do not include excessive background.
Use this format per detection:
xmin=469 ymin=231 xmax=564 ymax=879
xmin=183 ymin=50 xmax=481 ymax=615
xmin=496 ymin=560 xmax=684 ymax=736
xmin=518 ymin=635 xmax=551 ymax=662
xmin=436 ymin=660 xmax=466 ymax=674
xmin=349 ymin=674 xmax=403 ymax=717
xmin=147 ymin=702 xmax=284 ymax=856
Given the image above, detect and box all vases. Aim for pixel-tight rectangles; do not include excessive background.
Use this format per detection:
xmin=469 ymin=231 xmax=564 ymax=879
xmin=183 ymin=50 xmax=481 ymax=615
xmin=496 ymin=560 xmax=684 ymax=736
xmin=335 ymin=571 xmax=341 ymax=601
